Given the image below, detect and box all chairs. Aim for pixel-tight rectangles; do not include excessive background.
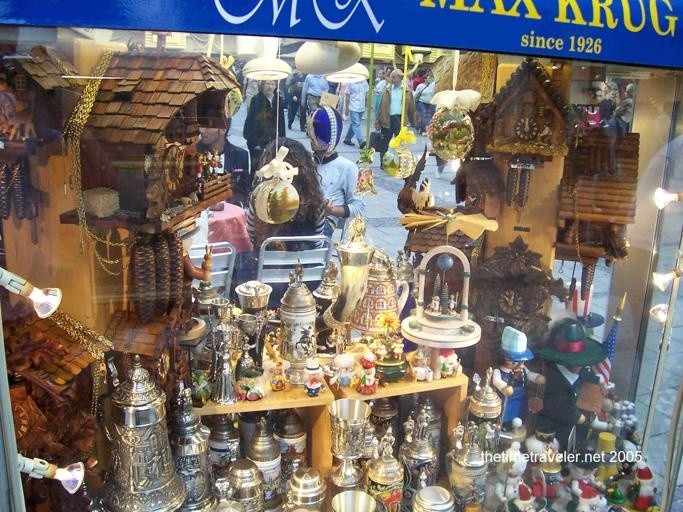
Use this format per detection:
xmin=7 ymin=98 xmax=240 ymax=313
xmin=255 ymin=235 xmax=334 ymax=309
xmin=188 ymin=241 xmax=236 ymax=304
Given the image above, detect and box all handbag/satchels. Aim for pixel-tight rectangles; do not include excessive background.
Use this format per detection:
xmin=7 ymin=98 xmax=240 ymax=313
xmin=368 ymin=130 xmax=382 ymax=153
xmin=415 ymin=91 xmax=422 ymax=102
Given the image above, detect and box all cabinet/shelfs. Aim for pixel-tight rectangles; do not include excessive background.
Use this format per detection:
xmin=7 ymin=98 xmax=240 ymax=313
xmin=177 ymin=356 xmax=470 ymax=512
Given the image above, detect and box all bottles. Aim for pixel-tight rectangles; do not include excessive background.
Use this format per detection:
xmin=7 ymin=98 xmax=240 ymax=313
xmin=93 ymin=256 xmax=520 ymax=511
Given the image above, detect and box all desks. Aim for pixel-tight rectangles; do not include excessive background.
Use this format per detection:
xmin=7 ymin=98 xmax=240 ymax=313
xmin=184 ymin=201 xmax=254 ymax=256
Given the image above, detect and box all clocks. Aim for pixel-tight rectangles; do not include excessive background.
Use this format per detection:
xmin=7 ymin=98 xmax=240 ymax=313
xmin=472 ymin=56 xmax=579 ymax=210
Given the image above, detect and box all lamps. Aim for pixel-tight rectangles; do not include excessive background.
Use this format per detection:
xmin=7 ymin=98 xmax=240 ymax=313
xmin=654 ymin=187 xmax=683 ymax=210
xmin=0 ymin=267 xmax=63 ymax=319
xmin=649 ymin=303 xmax=669 ymax=327
xmin=17 ymin=453 xmax=85 ymax=495
xmin=241 ymin=39 xmax=369 ymax=82
xmin=652 ymin=266 xmax=683 ymax=291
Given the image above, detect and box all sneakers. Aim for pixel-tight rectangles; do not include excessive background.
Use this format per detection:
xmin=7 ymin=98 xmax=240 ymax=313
xmin=343 ymin=139 xmax=355 ymax=146
xmin=359 ymin=141 xmax=366 ymax=148
xmin=288 ymin=124 xmax=307 ymax=131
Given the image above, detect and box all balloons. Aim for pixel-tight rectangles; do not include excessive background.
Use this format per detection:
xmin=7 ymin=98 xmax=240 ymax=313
xmin=492 ymin=326 xmax=547 ymax=431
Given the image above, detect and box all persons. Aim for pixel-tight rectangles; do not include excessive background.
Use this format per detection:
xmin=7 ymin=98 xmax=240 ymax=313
xmin=182 ymin=60 xmax=435 ymax=309
xmin=597 ymin=81 xmax=634 ymax=135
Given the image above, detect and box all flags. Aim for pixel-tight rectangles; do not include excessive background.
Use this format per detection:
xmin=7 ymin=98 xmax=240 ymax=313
xmin=591 ymin=322 xmax=618 ymax=383
xmin=583 ymin=284 xmax=593 ymax=316
xmin=566 ymin=287 xmax=577 ymax=316
